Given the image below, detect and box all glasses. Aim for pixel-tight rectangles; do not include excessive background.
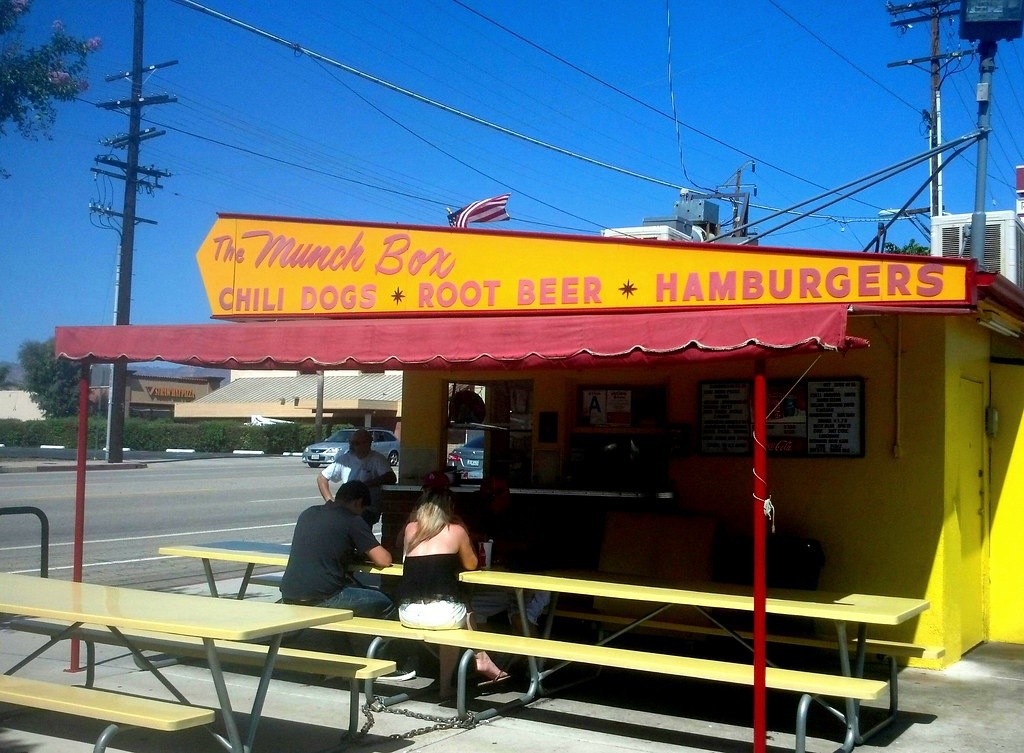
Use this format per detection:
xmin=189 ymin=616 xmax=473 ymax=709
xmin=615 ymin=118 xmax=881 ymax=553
xmin=349 ymin=440 xmax=367 ymax=446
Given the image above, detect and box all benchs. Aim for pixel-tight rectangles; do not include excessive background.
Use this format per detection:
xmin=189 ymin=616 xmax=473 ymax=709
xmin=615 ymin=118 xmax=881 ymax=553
xmin=1 ymin=674 xmax=216 ymax=753
xmin=8 ymin=618 xmax=396 ymax=742
xmin=543 ymin=610 xmax=945 ymax=718
xmin=310 ymin=617 xmax=887 ymax=752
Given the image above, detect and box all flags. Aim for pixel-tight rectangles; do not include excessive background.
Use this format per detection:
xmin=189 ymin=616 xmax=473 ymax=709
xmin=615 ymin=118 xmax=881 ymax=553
xmin=447 ymin=193 xmax=512 ymax=229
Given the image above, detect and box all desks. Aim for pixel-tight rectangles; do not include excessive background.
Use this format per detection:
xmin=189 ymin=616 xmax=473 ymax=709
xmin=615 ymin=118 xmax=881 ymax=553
xmin=159 ymin=545 xmax=930 ymax=742
xmin=0 ymin=573 xmax=354 ymax=753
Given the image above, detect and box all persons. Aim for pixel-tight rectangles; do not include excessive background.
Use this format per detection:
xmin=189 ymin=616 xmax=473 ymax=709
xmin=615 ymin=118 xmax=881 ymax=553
xmin=279 ymin=480 xmax=417 ymax=683
xmin=464 ymin=475 xmax=552 ymax=669
xmin=397 ymin=488 xmax=511 ymax=699
xmin=318 ymin=429 xmax=396 ymax=533
xmin=404 ymin=472 xmax=479 ymax=559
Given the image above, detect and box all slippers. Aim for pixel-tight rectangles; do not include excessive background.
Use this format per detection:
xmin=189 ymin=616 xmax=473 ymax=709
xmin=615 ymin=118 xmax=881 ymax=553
xmin=473 ymin=668 xmax=510 ymax=689
xmin=503 ymin=656 xmax=515 ymax=673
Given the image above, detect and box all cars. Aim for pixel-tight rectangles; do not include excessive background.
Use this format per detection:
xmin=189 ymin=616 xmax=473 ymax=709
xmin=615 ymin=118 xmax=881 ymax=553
xmin=447 ymin=436 xmax=485 ymax=480
xmin=301 ymin=428 xmax=401 ymax=473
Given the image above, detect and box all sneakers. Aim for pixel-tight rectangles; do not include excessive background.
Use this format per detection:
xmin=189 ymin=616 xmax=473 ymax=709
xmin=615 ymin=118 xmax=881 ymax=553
xmin=376 ymin=659 xmax=417 ymax=682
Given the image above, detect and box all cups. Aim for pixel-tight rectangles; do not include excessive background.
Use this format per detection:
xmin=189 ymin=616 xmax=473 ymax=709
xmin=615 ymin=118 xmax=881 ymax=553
xmin=477 ymin=542 xmax=492 ymax=570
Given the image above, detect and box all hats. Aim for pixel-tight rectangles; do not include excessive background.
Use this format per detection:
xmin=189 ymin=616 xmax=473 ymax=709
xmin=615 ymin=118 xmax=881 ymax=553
xmin=420 ymin=471 xmax=448 ymax=493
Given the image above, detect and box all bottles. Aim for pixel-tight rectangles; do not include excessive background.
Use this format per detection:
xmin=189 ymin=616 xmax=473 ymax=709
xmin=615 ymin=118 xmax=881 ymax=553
xmin=479 ymin=543 xmax=486 ymax=567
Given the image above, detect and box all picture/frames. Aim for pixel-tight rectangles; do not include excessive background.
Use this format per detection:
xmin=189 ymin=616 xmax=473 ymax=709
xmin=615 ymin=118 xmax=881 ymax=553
xmin=575 ymin=383 xmax=642 ymax=428
xmin=698 ymin=376 xmax=866 ymax=459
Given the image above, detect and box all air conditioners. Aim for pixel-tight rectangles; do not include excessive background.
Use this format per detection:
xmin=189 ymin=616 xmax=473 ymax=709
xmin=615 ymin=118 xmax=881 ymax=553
xmin=931 ymin=210 xmax=1024 ymax=292
